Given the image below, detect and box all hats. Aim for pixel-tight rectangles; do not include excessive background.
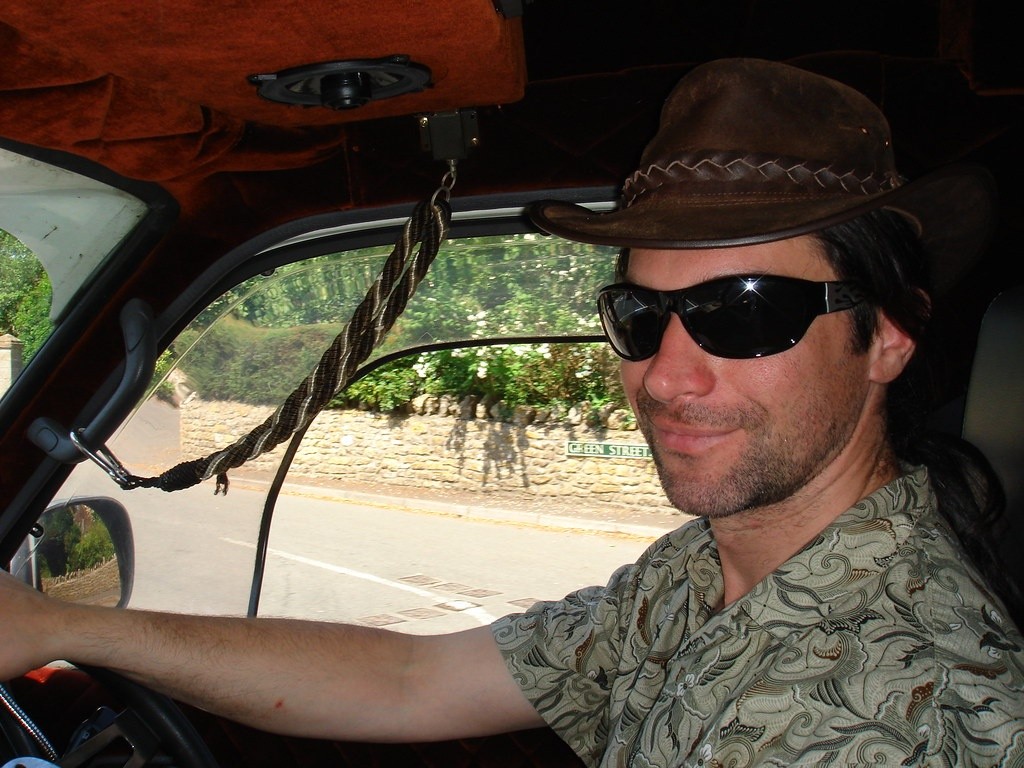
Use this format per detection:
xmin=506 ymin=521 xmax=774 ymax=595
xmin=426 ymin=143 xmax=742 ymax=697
xmin=525 ymin=62 xmax=989 ymax=282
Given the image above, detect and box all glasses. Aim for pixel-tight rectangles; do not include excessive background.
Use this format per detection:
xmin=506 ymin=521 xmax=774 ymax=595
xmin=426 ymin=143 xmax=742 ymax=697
xmin=597 ymin=260 xmax=897 ymax=361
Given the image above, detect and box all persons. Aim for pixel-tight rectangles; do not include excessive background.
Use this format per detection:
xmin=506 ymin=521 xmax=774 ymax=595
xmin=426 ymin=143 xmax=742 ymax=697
xmin=0 ymin=59 xmax=1024 ymax=768
